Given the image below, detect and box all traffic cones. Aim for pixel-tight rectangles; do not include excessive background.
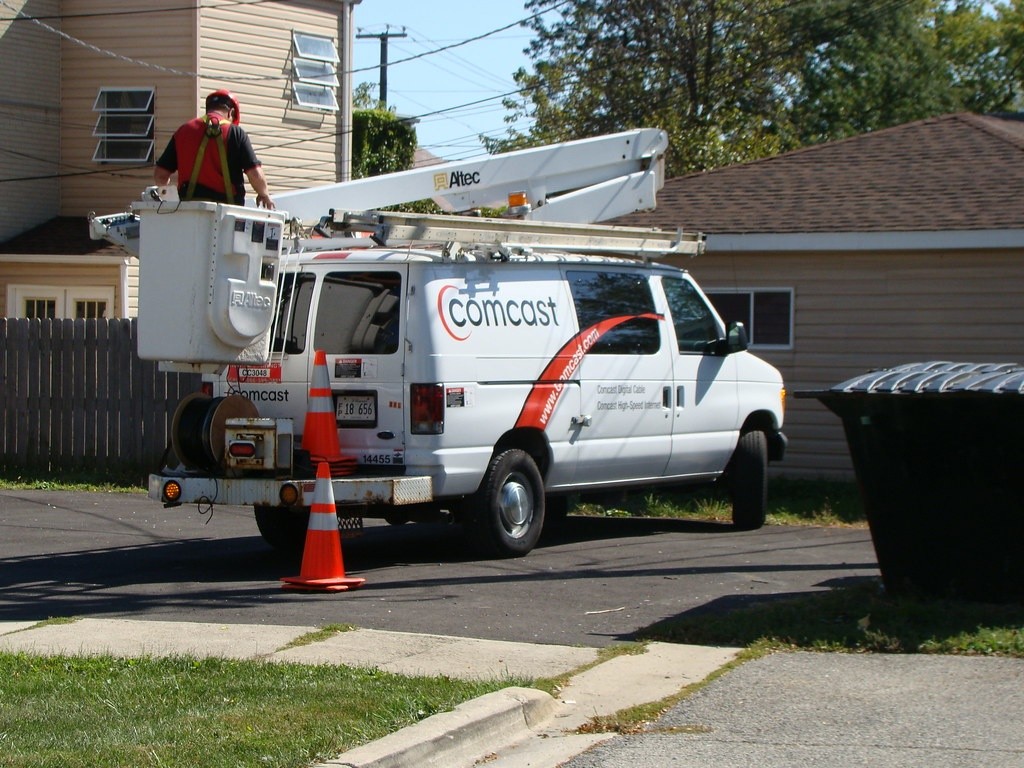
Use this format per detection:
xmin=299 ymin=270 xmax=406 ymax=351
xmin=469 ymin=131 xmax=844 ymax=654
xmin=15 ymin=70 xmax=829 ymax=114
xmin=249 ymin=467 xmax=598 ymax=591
xmin=301 ymin=348 xmax=357 ymax=479
xmin=278 ymin=460 xmax=366 ymax=593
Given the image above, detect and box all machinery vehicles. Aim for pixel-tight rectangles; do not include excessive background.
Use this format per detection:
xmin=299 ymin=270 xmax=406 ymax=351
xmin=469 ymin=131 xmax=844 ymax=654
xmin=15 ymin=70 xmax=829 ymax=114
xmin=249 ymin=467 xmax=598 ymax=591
xmin=82 ymin=125 xmax=791 ymax=560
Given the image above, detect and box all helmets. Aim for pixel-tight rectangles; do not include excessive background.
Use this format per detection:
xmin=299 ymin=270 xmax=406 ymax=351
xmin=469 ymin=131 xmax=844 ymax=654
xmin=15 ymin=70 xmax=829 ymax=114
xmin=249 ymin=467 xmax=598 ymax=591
xmin=206 ymin=90 xmax=240 ymax=127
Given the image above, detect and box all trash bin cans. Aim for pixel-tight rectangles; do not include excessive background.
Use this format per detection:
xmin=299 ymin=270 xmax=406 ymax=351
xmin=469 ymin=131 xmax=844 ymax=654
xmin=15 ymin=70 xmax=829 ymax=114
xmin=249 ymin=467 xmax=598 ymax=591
xmin=791 ymin=358 xmax=1024 ymax=601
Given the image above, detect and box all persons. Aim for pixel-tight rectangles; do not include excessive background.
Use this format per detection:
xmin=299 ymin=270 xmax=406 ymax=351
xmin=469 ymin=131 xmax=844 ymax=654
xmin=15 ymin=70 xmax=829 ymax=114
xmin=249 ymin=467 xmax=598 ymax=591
xmin=154 ymin=89 xmax=275 ymax=210
